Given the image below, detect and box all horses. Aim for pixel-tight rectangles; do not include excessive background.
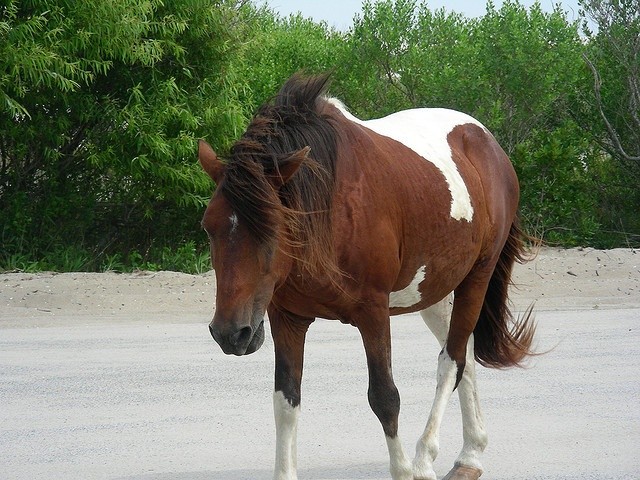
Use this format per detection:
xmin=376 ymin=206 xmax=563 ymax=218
xmin=197 ymin=67 xmax=567 ymax=480
